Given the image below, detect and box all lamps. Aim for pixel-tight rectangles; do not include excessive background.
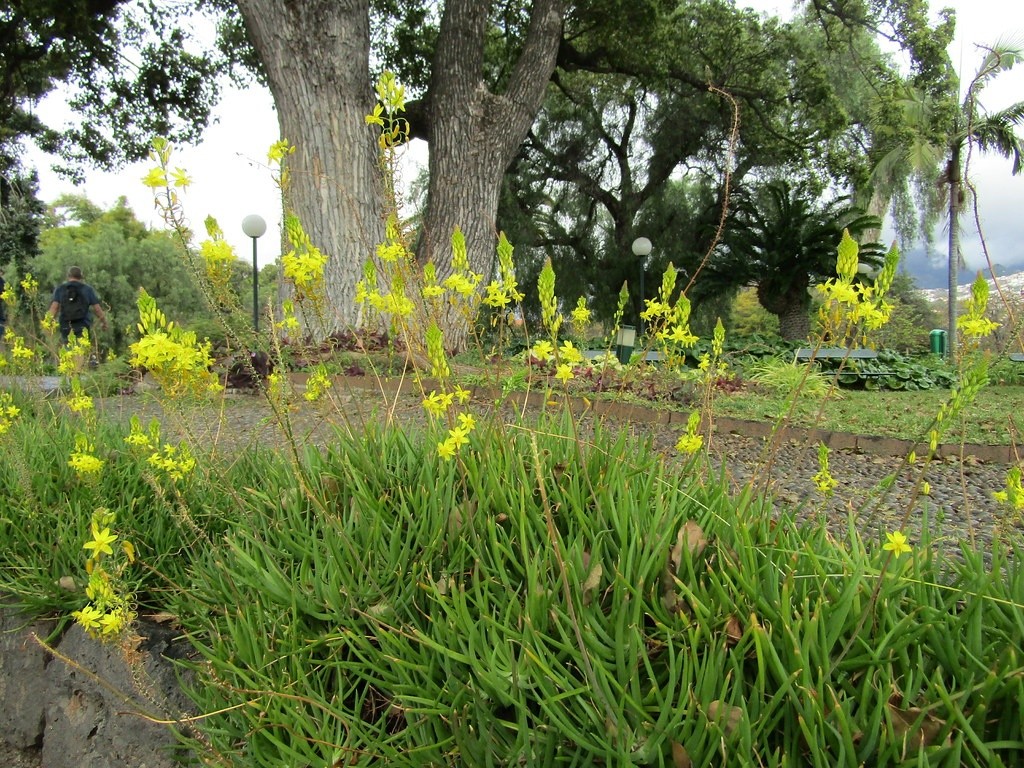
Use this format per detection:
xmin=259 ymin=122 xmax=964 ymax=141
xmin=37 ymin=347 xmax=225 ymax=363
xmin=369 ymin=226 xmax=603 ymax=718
xmin=617 ymin=324 xmax=636 ymax=348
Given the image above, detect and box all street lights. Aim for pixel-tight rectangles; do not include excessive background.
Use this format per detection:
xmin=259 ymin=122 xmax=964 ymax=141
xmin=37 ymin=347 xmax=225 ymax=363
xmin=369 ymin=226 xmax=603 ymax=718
xmin=631 ymin=237 xmax=653 ymax=352
xmin=241 ymin=213 xmax=269 ymax=350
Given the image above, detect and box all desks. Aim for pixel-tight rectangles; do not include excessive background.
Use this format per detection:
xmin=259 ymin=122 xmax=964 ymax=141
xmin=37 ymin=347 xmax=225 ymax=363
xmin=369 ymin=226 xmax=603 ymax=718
xmin=794 ymin=349 xmax=878 ymax=373
xmin=584 ymin=350 xmax=666 ymax=362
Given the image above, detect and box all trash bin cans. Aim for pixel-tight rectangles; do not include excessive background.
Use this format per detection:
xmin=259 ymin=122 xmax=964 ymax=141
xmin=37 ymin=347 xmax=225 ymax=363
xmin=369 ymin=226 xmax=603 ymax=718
xmin=930 ymin=329 xmax=948 ymax=358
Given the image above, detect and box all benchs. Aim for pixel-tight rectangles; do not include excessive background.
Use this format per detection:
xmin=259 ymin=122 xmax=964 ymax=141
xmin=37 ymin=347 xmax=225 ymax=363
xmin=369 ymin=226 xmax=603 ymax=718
xmin=825 ymin=372 xmax=897 ymax=393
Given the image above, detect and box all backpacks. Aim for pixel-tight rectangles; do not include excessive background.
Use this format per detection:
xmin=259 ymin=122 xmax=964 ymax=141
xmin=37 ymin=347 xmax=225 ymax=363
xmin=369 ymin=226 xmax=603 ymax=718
xmin=62 ymin=285 xmax=87 ymax=322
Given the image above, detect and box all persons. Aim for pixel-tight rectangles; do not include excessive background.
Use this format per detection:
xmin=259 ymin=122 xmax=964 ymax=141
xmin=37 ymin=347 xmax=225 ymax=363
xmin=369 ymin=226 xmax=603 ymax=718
xmin=43 ymin=265 xmax=109 ymax=349
